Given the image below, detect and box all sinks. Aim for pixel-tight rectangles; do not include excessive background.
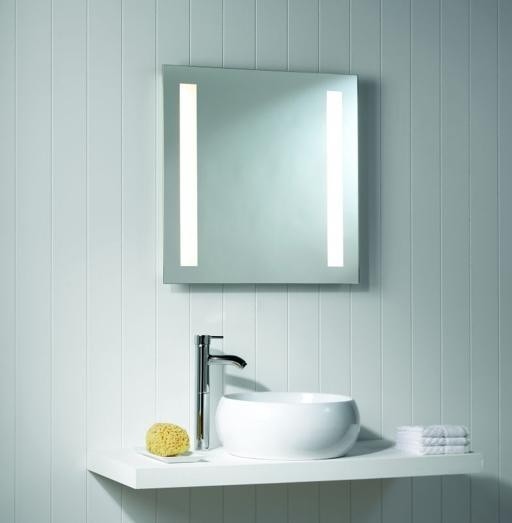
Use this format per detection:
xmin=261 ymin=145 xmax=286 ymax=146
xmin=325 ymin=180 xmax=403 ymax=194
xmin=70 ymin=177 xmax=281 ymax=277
xmin=214 ymin=392 xmax=362 ymax=460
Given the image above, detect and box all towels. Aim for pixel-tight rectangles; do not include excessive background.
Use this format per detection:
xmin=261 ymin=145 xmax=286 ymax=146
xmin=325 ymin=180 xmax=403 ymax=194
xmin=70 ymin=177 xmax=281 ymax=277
xmin=395 ymin=423 xmax=472 ymax=458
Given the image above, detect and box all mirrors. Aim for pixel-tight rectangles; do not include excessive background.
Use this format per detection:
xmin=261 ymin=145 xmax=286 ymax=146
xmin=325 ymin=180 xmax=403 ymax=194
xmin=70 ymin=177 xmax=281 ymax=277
xmin=153 ymin=60 xmax=362 ymax=288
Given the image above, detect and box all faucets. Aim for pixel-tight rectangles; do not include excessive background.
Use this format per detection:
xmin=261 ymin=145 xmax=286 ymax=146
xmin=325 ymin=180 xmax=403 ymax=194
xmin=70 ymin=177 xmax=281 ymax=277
xmin=192 ymin=334 xmax=248 ymax=449
